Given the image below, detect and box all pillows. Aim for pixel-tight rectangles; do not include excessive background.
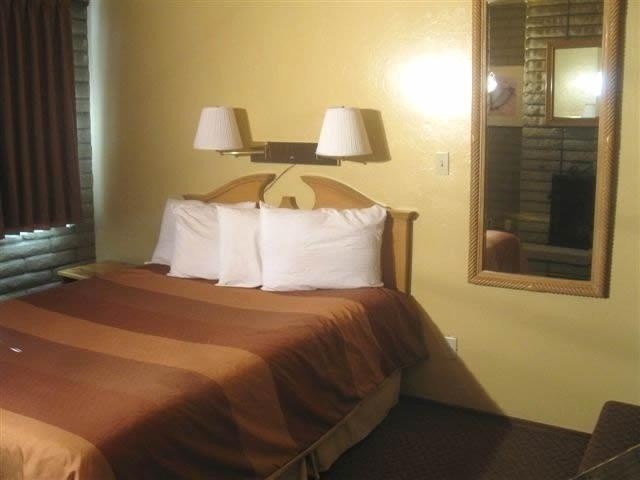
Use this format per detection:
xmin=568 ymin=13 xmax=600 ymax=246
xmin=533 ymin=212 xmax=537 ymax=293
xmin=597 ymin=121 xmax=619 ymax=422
xmin=147 ymin=195 xmax=391 ymax=296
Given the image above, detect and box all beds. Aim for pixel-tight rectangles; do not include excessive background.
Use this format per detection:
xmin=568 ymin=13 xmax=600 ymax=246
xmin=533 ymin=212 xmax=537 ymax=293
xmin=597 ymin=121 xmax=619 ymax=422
xmin=0 ymin=171 xmax=419 ymax=479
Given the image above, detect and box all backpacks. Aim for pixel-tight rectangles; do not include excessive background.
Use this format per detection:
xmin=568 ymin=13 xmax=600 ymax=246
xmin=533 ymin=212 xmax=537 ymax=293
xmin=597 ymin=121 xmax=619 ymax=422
xmin=549 ymin=169 xmax=596 ymax=250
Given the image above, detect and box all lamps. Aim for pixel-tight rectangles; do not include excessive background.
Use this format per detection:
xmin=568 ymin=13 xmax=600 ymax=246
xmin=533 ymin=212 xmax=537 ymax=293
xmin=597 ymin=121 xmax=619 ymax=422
xmin=316 ymin=105 xmax=373 ymax=162
xmin=191 ymin=105 xmax=269 ymax=163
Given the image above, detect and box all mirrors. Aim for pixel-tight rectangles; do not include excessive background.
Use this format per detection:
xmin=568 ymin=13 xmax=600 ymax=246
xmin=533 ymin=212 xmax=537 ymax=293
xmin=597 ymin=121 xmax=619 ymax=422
xmin=544 ymin=35 xmax=603 ymax=128
xmin=467 ymin=0 xmax=626 ymax=300
